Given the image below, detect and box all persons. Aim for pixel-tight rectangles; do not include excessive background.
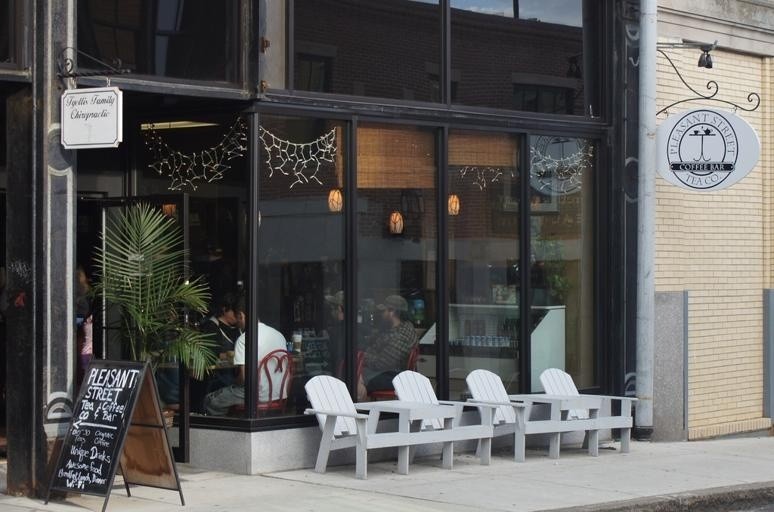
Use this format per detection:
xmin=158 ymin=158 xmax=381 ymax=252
xmin=324 ymin=289 xmax=346 ymax=358
xmin=82 ymin=309 xmax=93 ymax=381
xmin=203 ymin=295 xmax=293 ymax=417
xmin=356 ymin=293 xmax=419 ymax=403
xmin=75 ymin=265 xmax=94 ymax=330
xmin=201 ymin=290 xmax=244 ymax=393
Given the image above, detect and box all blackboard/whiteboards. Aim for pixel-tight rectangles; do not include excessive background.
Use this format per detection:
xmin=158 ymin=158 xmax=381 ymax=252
xmin=50 ymin=359 xmax=147 ymax=497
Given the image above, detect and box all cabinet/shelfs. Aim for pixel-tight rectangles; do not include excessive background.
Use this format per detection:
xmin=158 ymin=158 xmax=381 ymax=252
xmin=417 ymin=304 xmax=566 ymax=394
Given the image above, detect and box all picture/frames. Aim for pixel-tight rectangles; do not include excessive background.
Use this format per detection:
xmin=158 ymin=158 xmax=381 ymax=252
xmin=501 ymin=168 xmax=560 ymax=215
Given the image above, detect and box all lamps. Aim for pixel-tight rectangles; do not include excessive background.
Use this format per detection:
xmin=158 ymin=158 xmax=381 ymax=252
xmin=328 ymin=188 xmax=343 ymax=213
xmin=389 ymin=211 xmax=403 ymax=235
xmin=448 ymin=193 xmax=460 ymax=216
xmin=684 ymin=39 xmax=714 ymax=69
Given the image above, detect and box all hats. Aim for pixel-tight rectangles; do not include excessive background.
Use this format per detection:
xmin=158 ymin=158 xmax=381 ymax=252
xmin=375 ymin=293 xmax=408 ymax=313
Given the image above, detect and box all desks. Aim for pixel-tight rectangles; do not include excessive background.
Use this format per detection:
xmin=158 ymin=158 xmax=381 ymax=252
xmin=508 ymin=393 xmax=603 ymax=462
xmin=353 ymin=401 xmax=459 ymax=475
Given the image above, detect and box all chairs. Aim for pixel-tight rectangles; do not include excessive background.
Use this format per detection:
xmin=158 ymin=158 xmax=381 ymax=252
xmin=539 ymin=368 xmax=638 ymax=458
xmin=464 ymin=368 xmax=562 ymax=467
xmin=391 ymin=368 xmax=499 ymax=470
xmin=336 ymin=350 xmax=364 ymax=380
xmin=230 ymin=350 xmax=294 ymax=418
xmin=304 ymin=373 xmax=410 ymax=479
xmin=368 ymin=349 xmax=419 ymax=401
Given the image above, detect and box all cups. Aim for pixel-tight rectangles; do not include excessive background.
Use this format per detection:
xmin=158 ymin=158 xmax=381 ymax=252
xmin=227 ymin=351 xmax=234 ymax=364
xmin=287 ymin=334 xmax=302 ymax=352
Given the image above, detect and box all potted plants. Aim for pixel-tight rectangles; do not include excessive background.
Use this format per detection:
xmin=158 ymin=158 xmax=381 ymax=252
xmin=78 ymin=202 xmax=222 ymax=430
